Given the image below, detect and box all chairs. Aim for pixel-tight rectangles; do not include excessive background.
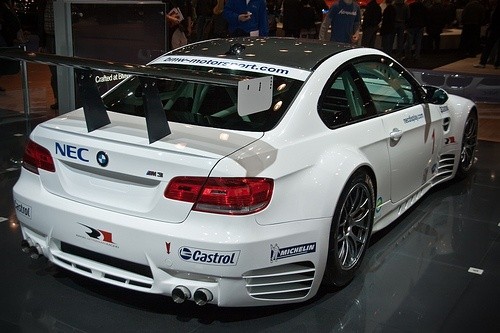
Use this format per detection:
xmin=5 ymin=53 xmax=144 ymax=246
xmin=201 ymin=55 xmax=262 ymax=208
xmin=197 ymin=82 xmax=351 ymax=133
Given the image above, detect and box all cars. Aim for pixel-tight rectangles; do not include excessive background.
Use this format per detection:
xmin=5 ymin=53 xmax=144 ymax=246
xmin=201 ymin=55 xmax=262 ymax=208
xmin=1 ymin=38 xmax=480 ymax=300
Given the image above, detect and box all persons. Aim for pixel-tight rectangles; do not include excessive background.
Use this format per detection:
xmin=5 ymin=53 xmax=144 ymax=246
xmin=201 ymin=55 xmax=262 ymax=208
xmin=361 ymin=0 xmax=500 ymax=72
xmin=165 ymin=0 xmax=318 ymax=51
xmin=0 ymin=0 xmax=65 ymax=111
xmin=319 ymin=0 xmax=361 ymax=46
